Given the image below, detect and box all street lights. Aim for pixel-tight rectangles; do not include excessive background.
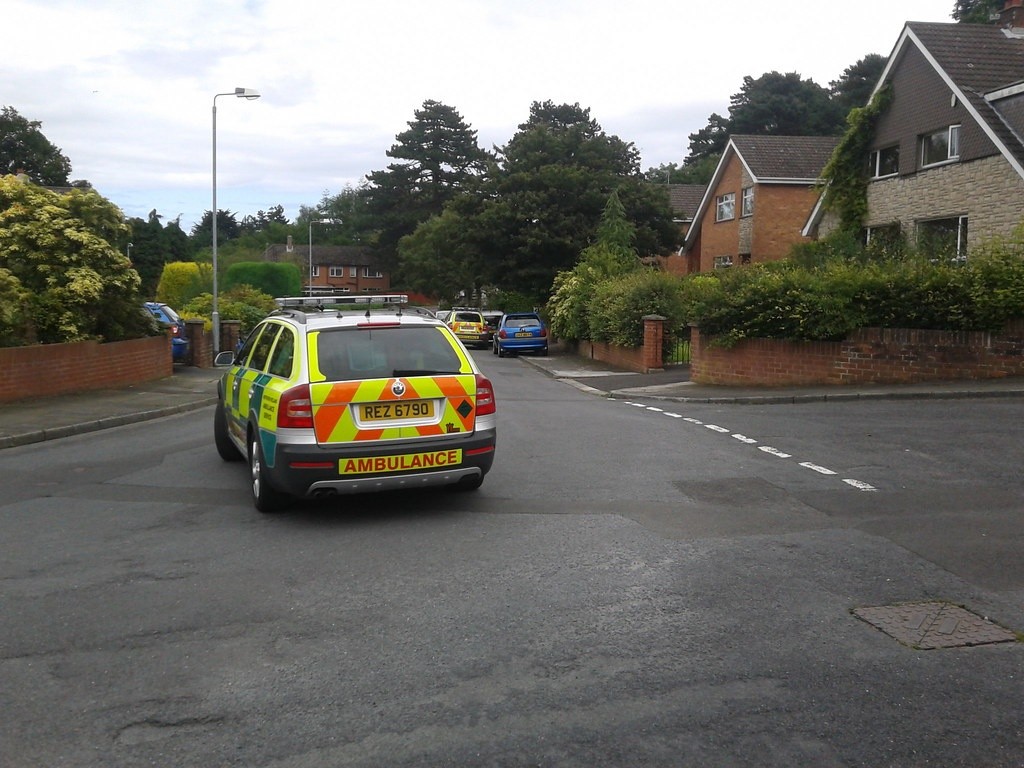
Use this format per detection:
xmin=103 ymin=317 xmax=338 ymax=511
xmin=127 ymin=243 xmax=133 ymax=258
xmin=309 ymin=218 xmax=343 ymax=296
xmin=213 ymin=88 xmax=261 ymax=367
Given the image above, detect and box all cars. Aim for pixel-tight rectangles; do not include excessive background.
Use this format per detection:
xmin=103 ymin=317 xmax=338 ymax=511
xmin=434 ymin=311 xmax=452 ymax=320
xmin=480 ymin=310 xmax=504 ymax=343
xmin=144 ymin=302 xmax=191 ymax=360
xmin=438 ymin=289 xmax=489 ymax=310
xmin=493 ymin=312 xmax=548 ymax=358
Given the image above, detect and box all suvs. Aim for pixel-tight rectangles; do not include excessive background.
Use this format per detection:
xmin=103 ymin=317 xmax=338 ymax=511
xmin=441 ymin=307 xmax=489 ymax=350
xmin=214 ymin=294 xmax=498 ymax=513
